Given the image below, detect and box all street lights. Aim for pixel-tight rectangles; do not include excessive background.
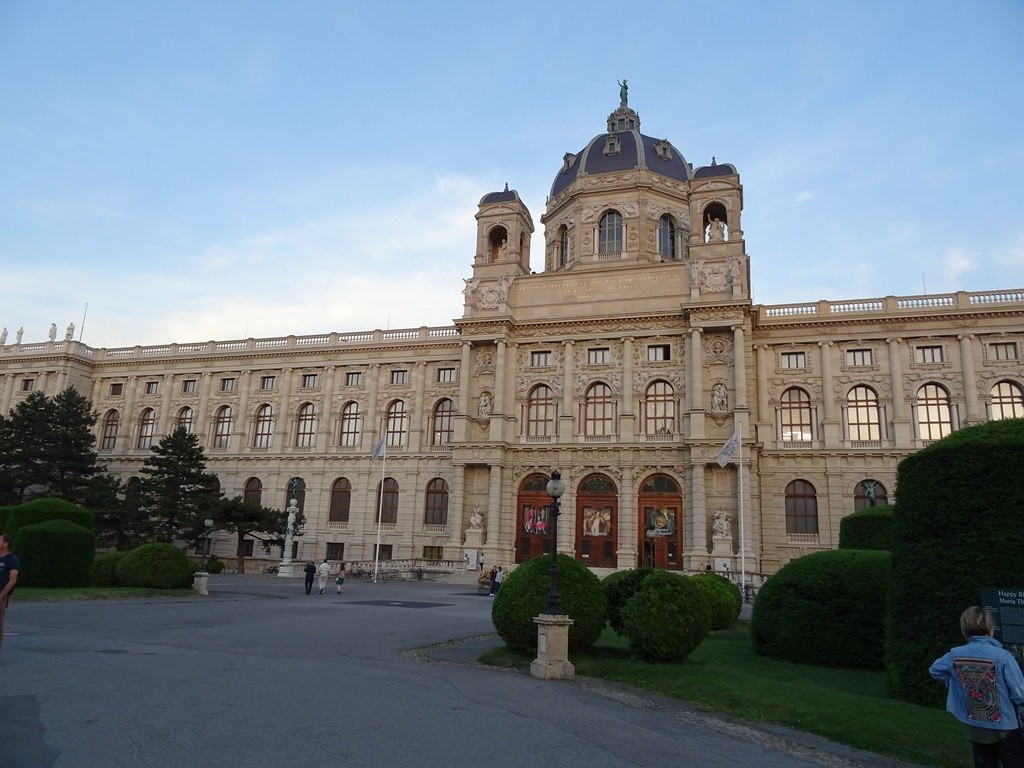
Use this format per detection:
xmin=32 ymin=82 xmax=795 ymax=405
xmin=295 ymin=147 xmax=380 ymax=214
xmin=544 ymin=467 xmax=566 ymax=616
xmin=198 ymin=512 xmax=214 ymax=572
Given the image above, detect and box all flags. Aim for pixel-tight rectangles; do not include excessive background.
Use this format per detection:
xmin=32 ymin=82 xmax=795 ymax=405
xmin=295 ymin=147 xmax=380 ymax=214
xmin=716 ymin=428 xmax=740 ymax=468
xmin=372 ymin=432 xmax=385 ymax=464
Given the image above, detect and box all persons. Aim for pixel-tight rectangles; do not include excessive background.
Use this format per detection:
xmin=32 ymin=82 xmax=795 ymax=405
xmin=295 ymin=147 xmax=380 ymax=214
xmin=49 ymin=323 xmax=57 ymax=341
xmin=290 ymin=479 xmax=298 ymax=499
xmin=489 ymin=565 xmax=503 ymax=597
xmin=650 ymin=204 xmax=660 ymax=215
xmin=477 ymin=393 xmax=490 ymax=417
xmin=705 ymin=182 xmax=720 ymax=189
xmin=479 ymin=552 xmax=485 ymax=571
xmin=499 ymin=276 xmax=508 ymax=300
xmin=303 ymin=560 xmax=345 ymax=595
xmin=692 ymin=260 xmax=700 ymax=284
xmin=699 ymin=560 xmax=729 ymax=576
xmin=729 ymin=256 xmax=740 ymax=280
xmin=866 ymin=484 xmax=877 ymax=504
xmin=679 ymin=211 xmax=689 ymax=229
xmin=712 ymin=384 xmax=728 ymax=412
xmin=590 ymin=510 xmax=605 ymax=535
xmin=525 ymin=506 xmax=546 ymax=533
xmin=584 ymin=204 xmax=604 ymax=220
xmin=708 ymin=214 xmax=725 ymax=239
xmin=501 ymin=238 xmax=508 ymax=258
xmin=462 ymin=279 xmax=474 ymax=303
xmin=470 ymin=502 xmax=485 ymax=531
xmin=64 ymin=322 xmax=75 ymax=341
xmin=616 ymin=202 xmax=636 ymax=215
xmin=17 ymin=327 xmax=24 ymax=344
xmin=483 ymin=354 xmax=685 ymax=391
xmin=712 ymin=505 xmax=732 ymax=537
xmin=0 ymin=534 xmax=20 ymax=647
xmin=929 ymin=606 xmax=1024 ymax=768
xmin=0 ymin=327 xmax=8 ymax=345
